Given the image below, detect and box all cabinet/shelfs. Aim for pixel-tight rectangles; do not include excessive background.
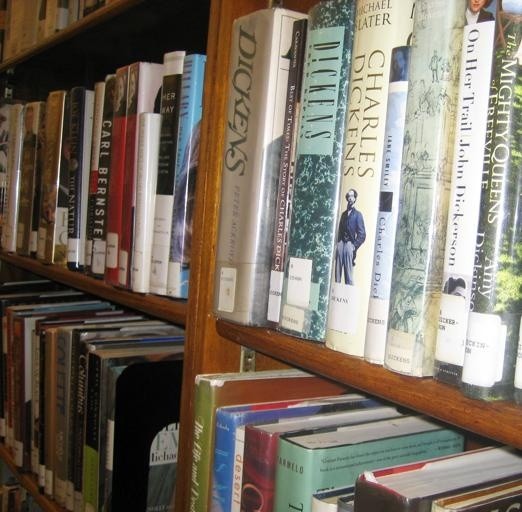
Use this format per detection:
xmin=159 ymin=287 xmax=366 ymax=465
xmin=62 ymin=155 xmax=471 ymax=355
xmin=0 ymin=0 xmax=522 ymax=510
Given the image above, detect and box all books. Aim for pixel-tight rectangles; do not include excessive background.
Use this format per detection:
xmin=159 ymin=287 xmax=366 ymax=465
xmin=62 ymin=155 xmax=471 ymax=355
xmin=2 ymin=0 xmax=522 ymax=512
